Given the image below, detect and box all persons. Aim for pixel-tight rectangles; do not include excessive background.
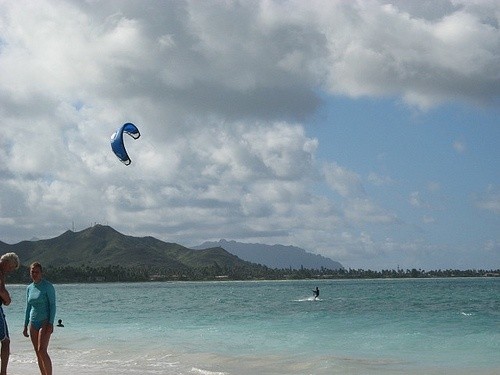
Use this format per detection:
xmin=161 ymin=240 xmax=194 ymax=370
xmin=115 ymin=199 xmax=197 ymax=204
xmin=312 ymin=287 xmax=319 ymax=299
xmin=56 ymin=319 xmax=64 ymax=327
xmin=23 ymin=263 xmax=56 ymax=375
xmin=0 ymin=252 xmax=20 ymax=375
